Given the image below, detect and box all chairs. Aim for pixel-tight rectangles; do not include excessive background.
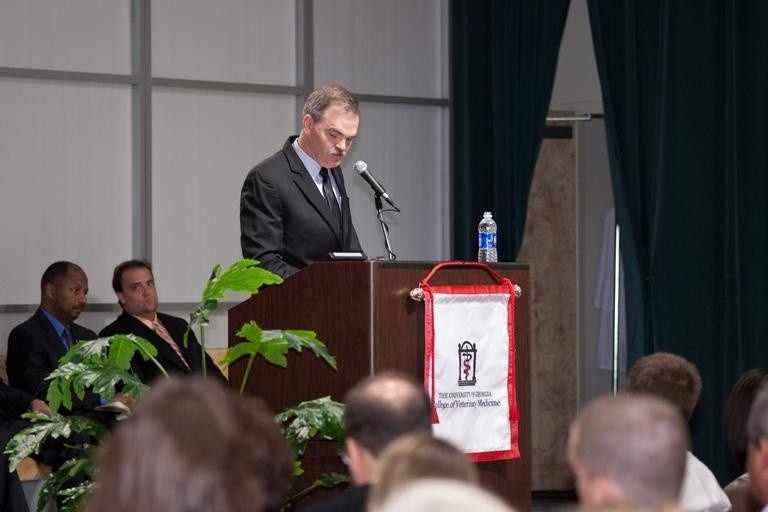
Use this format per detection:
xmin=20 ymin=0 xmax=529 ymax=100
xmin=0 ymin=345 xmax=230 ymax=487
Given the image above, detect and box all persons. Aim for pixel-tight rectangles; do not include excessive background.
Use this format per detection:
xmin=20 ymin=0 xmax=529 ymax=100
xmin=239 ymin=85 xmax=366 ymax=291
xmin=85 ymin=371 xmax=513 ymax=512
xmin=568 ymin=352 xmax=768 ymax=511
xmin=6 ymin=262 xmax=137 ymax=428
xmin=98 ymin=260 xmax=229 ymax=388
xmin=0 ymin=375 xmax=93 ymax=512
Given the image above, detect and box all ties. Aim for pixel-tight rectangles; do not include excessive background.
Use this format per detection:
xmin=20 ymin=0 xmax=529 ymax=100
xmin=318 ymin=166 xmax=346 ymax=232
xmin=60 ymin=326 xmax=77 ymax=349
xmin=149 ymin=321 xmax=193 ymax=374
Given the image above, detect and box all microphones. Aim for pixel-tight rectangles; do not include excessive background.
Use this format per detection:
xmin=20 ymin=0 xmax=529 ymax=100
xmin=351 ymin=160 xmax=399 ymax=211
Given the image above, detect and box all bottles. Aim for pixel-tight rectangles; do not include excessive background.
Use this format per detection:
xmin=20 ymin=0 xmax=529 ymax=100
xmin=478 ymin=211 xmax=498 ymax=263
